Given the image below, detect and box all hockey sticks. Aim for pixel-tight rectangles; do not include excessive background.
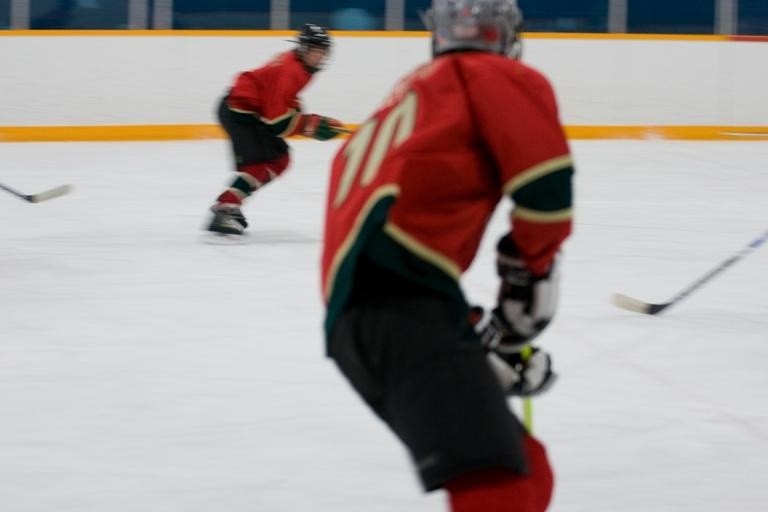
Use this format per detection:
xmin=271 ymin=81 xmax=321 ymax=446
xmin=612 ymin=233 xmax=764 ymax=316
xmin=0 ymin=181 xmax=69 ymax=204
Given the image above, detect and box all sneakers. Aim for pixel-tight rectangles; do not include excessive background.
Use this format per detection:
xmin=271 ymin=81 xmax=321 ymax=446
xmin=209 ymin=214 xmax=249 ymax=234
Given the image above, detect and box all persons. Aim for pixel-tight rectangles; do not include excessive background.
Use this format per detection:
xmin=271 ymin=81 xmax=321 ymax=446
xmin=316 ymin=2 xmax=577 ymax=511
xmin=207 ymin=21 xmax=346 ymax=234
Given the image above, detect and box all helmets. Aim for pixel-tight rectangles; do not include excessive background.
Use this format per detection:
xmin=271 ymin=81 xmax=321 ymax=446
xmin=300 ymin=23 xmax=331 ymax=50
xmin=431 ymin=0 xmax=524 ymax=60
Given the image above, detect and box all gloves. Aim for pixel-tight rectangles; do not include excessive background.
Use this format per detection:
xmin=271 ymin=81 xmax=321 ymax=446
xmin=481 ymin=335 xmax=558 ymax=398
xmin=478 ymin=232 xmax=562 ymax=355
xmin=300 ymin=114 xmax=345 ymax=142
